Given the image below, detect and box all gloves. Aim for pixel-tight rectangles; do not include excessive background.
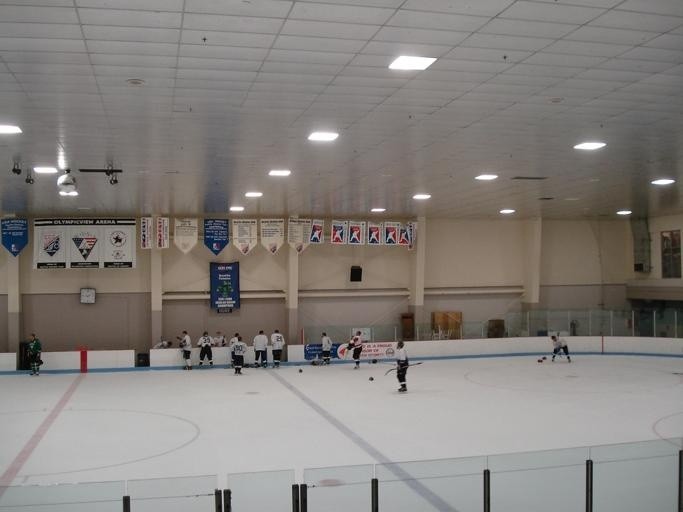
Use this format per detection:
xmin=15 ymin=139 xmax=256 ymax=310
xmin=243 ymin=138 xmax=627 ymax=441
xmin=397 ymin=365 xmax=400 ymax=370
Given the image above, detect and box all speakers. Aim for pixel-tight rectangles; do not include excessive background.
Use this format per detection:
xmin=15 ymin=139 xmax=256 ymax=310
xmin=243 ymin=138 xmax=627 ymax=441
xmin=350 ymin=266 xmax=363 ymax=282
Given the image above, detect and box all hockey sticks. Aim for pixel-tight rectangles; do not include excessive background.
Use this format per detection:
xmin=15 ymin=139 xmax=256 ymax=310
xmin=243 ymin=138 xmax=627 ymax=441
xmin=385 ymin=363 xmax=423 ymax=376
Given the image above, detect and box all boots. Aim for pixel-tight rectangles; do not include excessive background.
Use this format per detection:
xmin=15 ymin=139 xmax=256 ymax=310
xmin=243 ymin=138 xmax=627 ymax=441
xmin=399 ymin=384 xmax=407 ymax=391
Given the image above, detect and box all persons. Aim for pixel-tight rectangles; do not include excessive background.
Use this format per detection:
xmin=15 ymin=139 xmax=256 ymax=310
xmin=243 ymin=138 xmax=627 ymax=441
xmin=551 ymin=336 xmax=572 ymax=363
xmin=395 ymin=341 xmax=410 ymax=392
xmin=270 ymin=330 xmax=285 ymax=368
xmin=27 ymin=334 xmax=41 ymax=375
xmin=179 ymin=331 xmax=193 ymax=370
xmin=253 ymin=330 xmax=269 ymax=367
xmin=153 ymin=341 xmax=172 ymax=348
xmin=229 ymin=333 xmax=240 ymax=362
xmin=349 ymin=331 xmax=363 ymax=369
xmin=320 ymin=332 xmax=333 ymax=365
xmin=196 ymin=331 xmax=215 ymax=369
xmin=214 ymin=331 xmax=227 ymax=347
xmin=230 ymin=337 xmax=249 ymax=374
